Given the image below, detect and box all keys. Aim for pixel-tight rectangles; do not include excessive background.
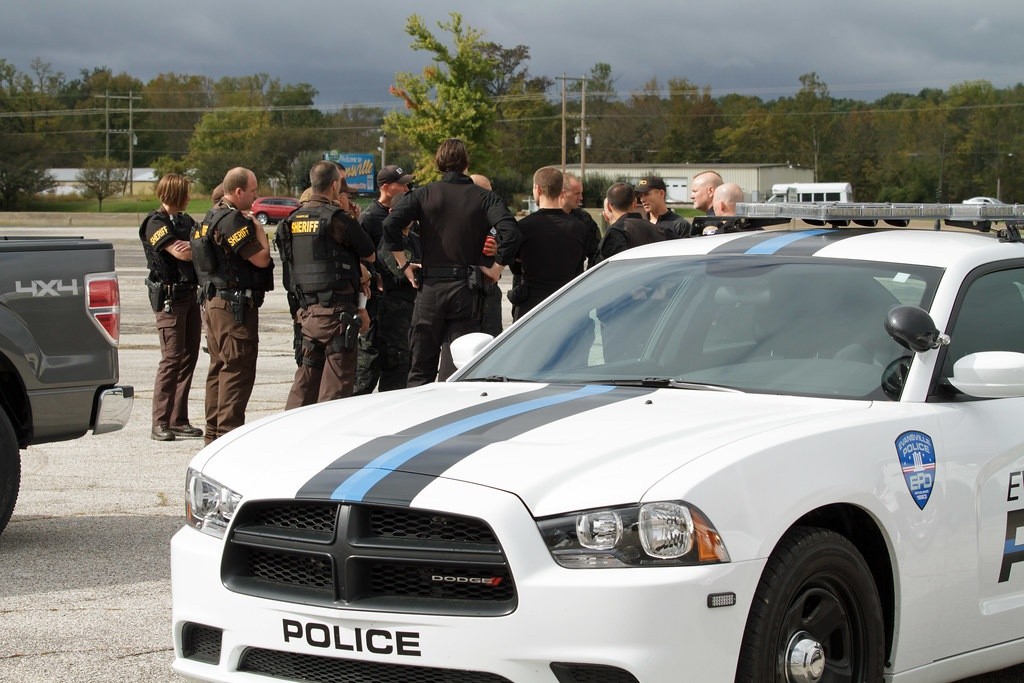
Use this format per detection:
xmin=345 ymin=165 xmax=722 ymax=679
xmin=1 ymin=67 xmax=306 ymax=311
xmin=164 ymin=299 xmax=172 ymax=312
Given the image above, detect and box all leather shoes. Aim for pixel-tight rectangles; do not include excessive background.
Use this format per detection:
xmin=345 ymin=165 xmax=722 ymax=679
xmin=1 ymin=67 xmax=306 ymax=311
xmin=150 ymin=429 xmax=176 ymax=441
xmin=170 ymin=424 xmax=203 ymax=436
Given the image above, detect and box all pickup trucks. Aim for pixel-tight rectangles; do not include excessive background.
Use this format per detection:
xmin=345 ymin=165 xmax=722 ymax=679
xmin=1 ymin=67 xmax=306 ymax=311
xmin=0 ymin=234 xmax=135 ymax=535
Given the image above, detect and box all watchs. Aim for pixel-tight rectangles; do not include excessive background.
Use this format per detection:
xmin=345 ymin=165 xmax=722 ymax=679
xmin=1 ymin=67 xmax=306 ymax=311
xmin=397 ymin=259 xmax=410 ymax=274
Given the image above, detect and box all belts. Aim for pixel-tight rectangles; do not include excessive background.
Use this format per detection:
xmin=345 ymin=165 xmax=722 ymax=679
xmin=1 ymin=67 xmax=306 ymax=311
xmin=420 ymin=266 xmax=470 ymax=277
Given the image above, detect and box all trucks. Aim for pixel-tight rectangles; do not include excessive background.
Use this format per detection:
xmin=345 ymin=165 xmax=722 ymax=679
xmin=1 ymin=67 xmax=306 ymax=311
xmin=766 ymin=183 xmax=853 ymax=204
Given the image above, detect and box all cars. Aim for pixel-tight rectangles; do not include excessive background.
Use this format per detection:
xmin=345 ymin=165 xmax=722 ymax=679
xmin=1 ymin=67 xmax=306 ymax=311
xmin=250 ymin=196 xmax=302 ymax=225
xmin=962 ymin=196 xmax=1005 ymax=205
xmin=170 ymin=199 xmax=1024 ymax=683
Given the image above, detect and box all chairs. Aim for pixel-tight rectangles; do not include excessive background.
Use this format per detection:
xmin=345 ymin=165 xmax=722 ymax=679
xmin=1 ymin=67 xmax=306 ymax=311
xmin=740 ymin=288 xmax=876 ymax=365
xmin=941 ymin=277 xmax=1024 ymax=378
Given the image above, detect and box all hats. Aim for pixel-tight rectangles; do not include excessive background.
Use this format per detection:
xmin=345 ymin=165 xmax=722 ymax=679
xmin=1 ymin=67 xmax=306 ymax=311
xmin=377 ymin=164 xmax=415 ymax=186
xmin=339 ymin=175 xmax=358 ymax=193
xmin=636 ymin=175 xmax=666 ymax=193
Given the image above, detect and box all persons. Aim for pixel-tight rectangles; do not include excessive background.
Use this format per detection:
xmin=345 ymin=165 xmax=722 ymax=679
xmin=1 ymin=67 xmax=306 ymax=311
xmin=275 ymin=137 xmax=763 ymax=411
xmin=139 ymin=167 xmax=270 ymax=448
